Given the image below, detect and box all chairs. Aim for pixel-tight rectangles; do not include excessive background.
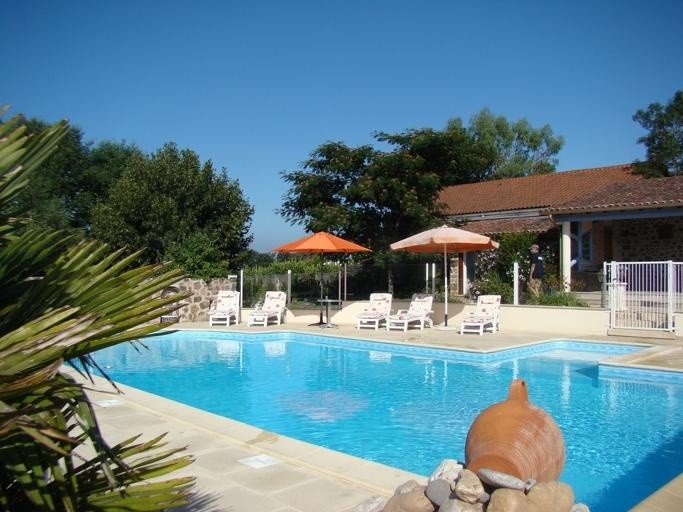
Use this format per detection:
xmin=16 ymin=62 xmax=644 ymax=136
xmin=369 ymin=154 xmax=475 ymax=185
xmin=210 ymin=291 xmax=241 ymax=327
xmin=357 ymin=292 xmax=392 ymax=331
xmin=248 ymin=291 xmax=287 ymax=327
xmin=387 ymin=293 xmax=436 ymax=333
xmin=460 ymin=295 xmax=502 ymax=335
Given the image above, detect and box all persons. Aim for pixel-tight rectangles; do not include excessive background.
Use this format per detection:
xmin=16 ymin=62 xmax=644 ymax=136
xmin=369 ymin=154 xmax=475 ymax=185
xmin=525 ymin=242 xmax=544 ymax=298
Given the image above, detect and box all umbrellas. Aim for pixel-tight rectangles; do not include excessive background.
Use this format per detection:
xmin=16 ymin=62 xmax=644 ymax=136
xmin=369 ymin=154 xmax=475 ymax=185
xmin=389 ymin=224 xmax=501 ymax=315
xmin=270 ymin=231 xmax=373 ymax=313
